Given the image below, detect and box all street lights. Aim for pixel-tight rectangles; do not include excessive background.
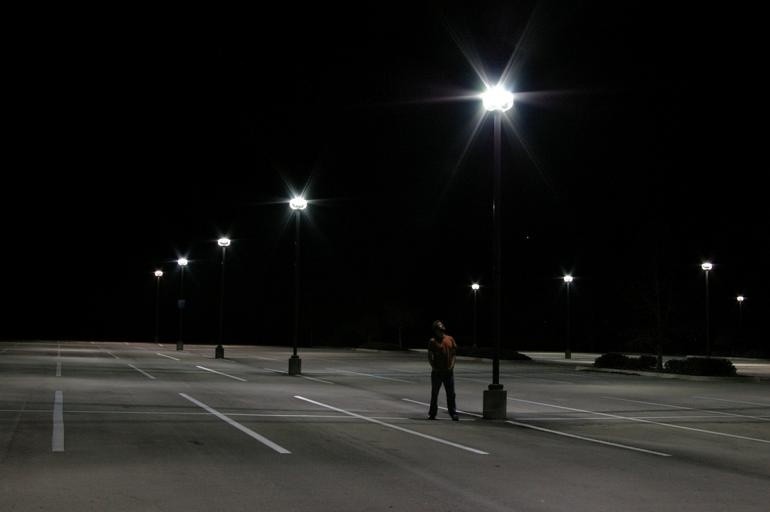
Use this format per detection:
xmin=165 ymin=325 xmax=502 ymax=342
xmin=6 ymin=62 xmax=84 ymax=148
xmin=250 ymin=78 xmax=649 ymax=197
xmin=563 ymin=274 xmax=574 ymax=359
xmin=470 ymin=283 xmax=481 ymax=356
xmin=175 ymin=256 xmax=189 ymax=350
xmin=213 ymin=237 xmax=230 ymax=359
xmin=700 ymin=258 xmax=713 ymax=355
xmin=478 ymin=82 xmax=515 ymax=419
xmin=286 ymin=195 xmax=309 ymax=376
xmin=154 ymin=269 xmax=164 ymax=344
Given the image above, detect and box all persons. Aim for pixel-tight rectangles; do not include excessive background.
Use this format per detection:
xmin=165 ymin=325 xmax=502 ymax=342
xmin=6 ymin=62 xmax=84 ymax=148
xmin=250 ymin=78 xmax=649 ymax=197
xmin=427 ymin=320 xmax=459 ymax=421
